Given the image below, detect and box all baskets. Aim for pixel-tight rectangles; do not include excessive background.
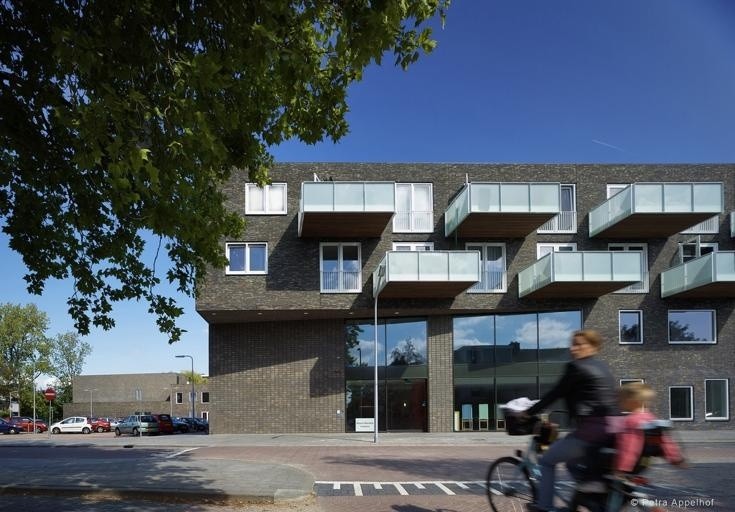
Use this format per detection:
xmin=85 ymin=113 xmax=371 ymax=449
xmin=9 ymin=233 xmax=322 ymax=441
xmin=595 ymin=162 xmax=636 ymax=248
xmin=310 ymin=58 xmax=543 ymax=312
xmin=503 ymin=407 xmax=548 ymax=436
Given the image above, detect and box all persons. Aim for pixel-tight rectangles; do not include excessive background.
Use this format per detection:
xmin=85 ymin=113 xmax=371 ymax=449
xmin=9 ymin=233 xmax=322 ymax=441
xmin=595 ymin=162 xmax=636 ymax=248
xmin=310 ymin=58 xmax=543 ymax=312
xmin=505 ymin=329 xmax=621 ymax=512
xmin=576 ymin=383 xmax=688 ymax=512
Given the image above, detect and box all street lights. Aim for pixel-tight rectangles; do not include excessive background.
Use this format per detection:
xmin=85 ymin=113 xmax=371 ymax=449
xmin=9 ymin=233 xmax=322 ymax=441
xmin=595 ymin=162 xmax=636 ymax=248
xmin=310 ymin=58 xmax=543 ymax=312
xmin=373 ymin=264 xmax=386 ymax=442
xmin=175 ymin=354 xmax=194 ymax=431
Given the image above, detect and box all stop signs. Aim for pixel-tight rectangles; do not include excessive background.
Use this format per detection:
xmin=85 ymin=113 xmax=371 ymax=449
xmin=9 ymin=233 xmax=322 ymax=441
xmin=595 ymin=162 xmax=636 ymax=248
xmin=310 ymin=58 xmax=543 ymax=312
xmin=45 ymin=387 xmax=56 ymax=401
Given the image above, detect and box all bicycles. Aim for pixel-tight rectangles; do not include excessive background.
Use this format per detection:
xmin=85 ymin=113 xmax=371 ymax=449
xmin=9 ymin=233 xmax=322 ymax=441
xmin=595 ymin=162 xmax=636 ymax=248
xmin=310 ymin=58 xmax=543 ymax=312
xmin=487 ymin=409 xmax=665 ymax=511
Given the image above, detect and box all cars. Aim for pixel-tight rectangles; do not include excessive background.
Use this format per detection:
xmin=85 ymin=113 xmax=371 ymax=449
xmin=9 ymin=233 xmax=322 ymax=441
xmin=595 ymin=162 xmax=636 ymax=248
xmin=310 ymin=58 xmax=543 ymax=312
xmin=0 ymin=417 xmax=23 ymax=434
xmin=49 ymin=416 xmax=92 ymax=434
xmin=88 ymin=413 xmax=208 ymax=436
xmin=5 ymin=416 xmax=48 ymax=434
